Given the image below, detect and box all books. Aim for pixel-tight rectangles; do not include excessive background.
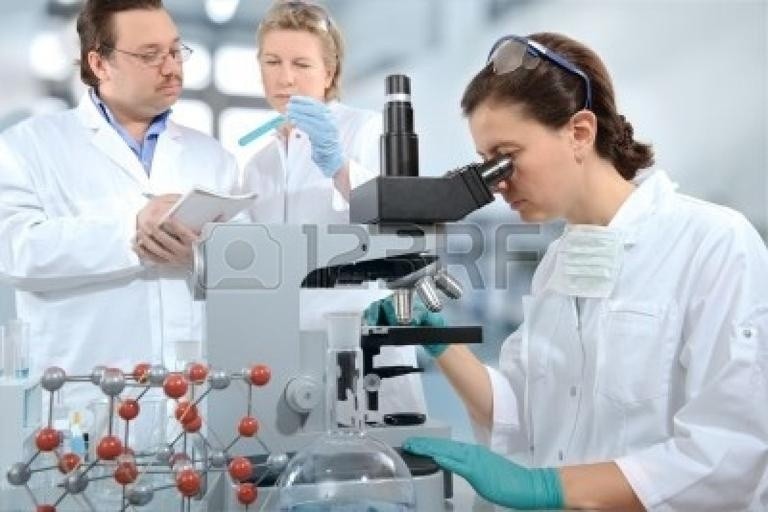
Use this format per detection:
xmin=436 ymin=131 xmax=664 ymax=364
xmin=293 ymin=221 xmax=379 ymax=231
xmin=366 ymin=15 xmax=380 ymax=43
xmin=138 ymin=187 xmax=257 ymax=247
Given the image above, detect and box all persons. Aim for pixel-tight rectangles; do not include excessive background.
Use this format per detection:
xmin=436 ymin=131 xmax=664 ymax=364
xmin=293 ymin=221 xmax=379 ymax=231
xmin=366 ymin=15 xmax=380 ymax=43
xmin=246 ymin=1 xmax=386 ymax=332
xmin=359 ymin=32 xmax=768 ymax=510
xmin=0 ymin=2 xmax=241 ymax=476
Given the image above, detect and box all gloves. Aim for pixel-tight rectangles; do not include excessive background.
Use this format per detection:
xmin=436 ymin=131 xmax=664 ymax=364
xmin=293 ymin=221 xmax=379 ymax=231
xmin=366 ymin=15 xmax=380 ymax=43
xmin=285 ymin=95 xmax=347 ymax=178
xmin=362 ymin=293 xmax=450 ymax=357
xmin=402 ymin=433 xmax=564 ymax=511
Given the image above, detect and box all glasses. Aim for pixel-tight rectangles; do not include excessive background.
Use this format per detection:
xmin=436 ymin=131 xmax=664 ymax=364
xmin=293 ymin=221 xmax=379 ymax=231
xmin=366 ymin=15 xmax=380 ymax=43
xmin=89 ymin=40 xmax=194 ymax=65
xmin=486 ymin=35 xmax=592 ymax=110
